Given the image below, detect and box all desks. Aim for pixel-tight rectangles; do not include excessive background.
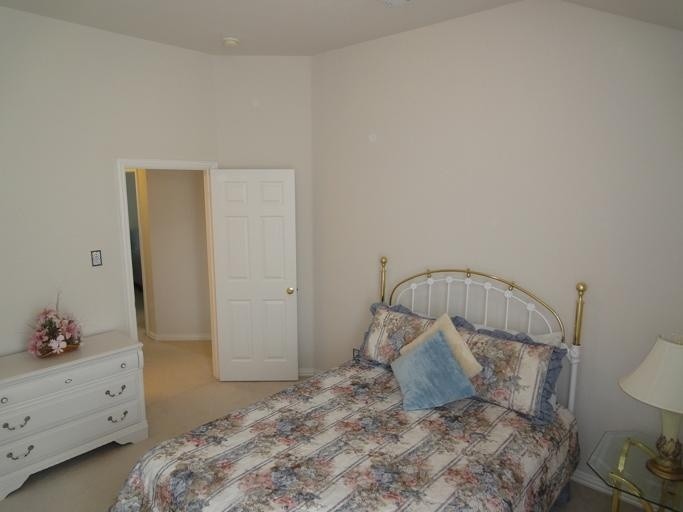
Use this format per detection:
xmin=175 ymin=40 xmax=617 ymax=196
xmin=585 ymin=427 xmax=682 ymax=506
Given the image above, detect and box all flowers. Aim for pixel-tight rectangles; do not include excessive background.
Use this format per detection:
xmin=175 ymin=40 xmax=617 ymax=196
xmin=20 ymin=302 xmax=91 ymax=360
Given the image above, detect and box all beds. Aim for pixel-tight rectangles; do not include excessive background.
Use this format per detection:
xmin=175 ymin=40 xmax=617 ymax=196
xmin=109 ymin=252 xmax=600 ymax=507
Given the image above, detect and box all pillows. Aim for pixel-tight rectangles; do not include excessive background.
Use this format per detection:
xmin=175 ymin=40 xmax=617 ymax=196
xmin=359 ymin=304 xmax=569 ymax=427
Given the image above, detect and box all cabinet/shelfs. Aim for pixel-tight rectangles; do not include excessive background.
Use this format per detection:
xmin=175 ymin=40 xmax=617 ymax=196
xmin=2 ymin=330 xmax=154 ymax=502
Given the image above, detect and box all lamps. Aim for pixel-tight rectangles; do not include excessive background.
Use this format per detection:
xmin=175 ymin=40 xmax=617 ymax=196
xmin=614 ymin=332 xmax=683 ymax=480
